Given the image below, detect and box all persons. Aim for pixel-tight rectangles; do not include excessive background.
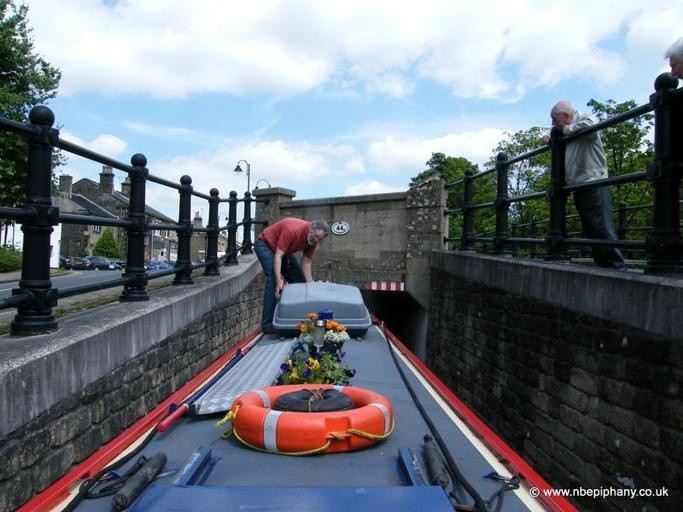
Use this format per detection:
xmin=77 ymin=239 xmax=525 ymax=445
xmin=663 ymin=31 xmax=682 ymax=83
xmin=540 ymin=100 xmax=624 ymax=272
xmin=251 ymin=217 xmax=329 ymax=332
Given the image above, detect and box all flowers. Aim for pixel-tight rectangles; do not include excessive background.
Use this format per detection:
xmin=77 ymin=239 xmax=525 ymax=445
xmin=275 ymin=349 xmax=356 ymax=385
xmin=292 ymin=312 xmax=351 ymax=347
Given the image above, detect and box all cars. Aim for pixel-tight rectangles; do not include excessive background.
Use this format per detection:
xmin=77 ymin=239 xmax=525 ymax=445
xmin=60 ymin=255 xmax=175 ymax=271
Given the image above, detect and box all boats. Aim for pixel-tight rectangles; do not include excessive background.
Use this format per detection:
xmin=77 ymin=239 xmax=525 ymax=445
xmin=13 ymin=281 xmax=580 ymax=511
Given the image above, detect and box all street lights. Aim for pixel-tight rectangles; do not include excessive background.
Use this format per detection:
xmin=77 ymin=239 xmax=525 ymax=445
xmin=255 ymin=178 xmax=271 ymax=189
xmin=233 ymin=159 xmax=252 ymax=195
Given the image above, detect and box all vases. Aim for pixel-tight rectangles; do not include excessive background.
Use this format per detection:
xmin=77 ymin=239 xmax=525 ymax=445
xmin=308 ymin=342 xmax=337 ymax=358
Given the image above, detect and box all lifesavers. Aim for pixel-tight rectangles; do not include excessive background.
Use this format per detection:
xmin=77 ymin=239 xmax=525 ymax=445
xmin=216 ymin=384 xmax=395 ymax=456
